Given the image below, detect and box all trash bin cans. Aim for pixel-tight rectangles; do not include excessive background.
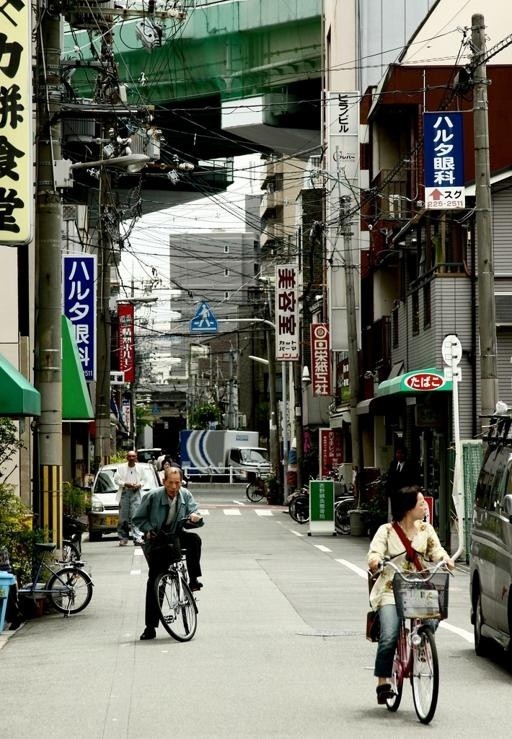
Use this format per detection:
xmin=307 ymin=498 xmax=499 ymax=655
xmin=349 ymin=511 xmax=369 ymax=537
xmin=0 ymin=571 xmax=16 ymax=633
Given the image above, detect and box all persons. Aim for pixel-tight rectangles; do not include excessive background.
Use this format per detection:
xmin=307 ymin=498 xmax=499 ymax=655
xmin=382 ymin=444 xmax=416 ymax=519
xmin=116 ymin=450 xmax=148 ymax=546
xmin=368 ymin=487 xmax=455 ymax=704
xmin=131 ymin=467 xmax=204 ymax=639
xmin=148 ymin=450 xmax=188 ymax=486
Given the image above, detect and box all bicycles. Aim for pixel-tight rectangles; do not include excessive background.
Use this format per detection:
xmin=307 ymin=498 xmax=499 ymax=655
xmin=147 ymin=517 xmax=202 ymax=642
xmin=373 ymin=556 xmax=452 ymax=724
xmin=290 ymin=469 xmax=389 ymax=536
xmin=1 ymin=538 xmax=94 ymax=618
xmin=245 ymin=477 xmax=268 ymax=503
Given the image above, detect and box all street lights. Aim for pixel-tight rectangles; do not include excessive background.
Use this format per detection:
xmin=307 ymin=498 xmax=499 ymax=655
xmin=35 ymin=153 xmax=152 ymax=615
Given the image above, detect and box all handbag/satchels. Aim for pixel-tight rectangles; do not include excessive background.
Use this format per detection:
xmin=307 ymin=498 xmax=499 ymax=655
xmin=425 ymin=583 xmax=442 ymax=616
xmin=367 ymin=611 xmax=380 ymax=642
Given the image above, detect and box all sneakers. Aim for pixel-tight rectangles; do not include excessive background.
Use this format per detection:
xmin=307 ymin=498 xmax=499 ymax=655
xmin=377 ymin=684 xmax=391 ymax=704
xmin=120 ymin=540 xmax=142 ymax=546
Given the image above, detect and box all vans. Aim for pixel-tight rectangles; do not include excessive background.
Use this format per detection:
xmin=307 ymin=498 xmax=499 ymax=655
xmin=469 ymin=412 xmax=512 ymax=671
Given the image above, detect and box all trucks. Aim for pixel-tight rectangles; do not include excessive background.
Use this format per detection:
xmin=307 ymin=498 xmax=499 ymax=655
xmin=179 ymin=429 xmax=275 ymax=483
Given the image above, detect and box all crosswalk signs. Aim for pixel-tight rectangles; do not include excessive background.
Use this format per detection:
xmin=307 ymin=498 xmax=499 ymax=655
xmin=190 ymin=301 xmax=219 ymax=332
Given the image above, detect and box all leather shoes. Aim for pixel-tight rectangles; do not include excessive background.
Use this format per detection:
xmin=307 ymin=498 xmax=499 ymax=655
xmin=188 ymin=581 xmax=203 ymax=592
xmin=140 ymin=628 xmax=156 ymax=640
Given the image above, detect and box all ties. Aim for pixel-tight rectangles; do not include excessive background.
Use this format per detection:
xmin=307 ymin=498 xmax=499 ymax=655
xmin=397 ymin=464 xmax=400 ymax=472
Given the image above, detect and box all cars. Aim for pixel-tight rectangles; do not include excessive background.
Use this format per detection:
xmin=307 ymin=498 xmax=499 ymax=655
xmin=87 ymin=462 xmax=163 ymax=542
xmin=137 ymin=448 xmax=186 ymax=487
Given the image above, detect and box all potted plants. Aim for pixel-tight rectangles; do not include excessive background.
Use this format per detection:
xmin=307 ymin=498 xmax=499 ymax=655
xmin=16 ymin=527 xmax=54 ymax=617
